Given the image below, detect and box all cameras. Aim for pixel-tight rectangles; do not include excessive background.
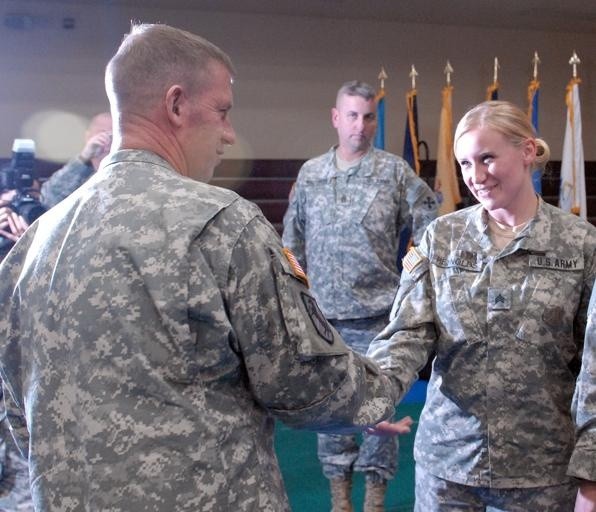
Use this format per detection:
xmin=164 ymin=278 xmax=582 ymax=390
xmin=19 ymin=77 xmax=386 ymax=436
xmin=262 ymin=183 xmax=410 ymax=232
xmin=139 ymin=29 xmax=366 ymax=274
xmin=0 ymin=151 xmax=47 ymax=251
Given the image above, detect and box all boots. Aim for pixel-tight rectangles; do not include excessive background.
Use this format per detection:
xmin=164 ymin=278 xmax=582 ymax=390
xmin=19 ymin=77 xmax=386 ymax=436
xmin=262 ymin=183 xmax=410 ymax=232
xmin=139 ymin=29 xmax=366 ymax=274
xmin=328 ymin=476 xmax=353 ymax=512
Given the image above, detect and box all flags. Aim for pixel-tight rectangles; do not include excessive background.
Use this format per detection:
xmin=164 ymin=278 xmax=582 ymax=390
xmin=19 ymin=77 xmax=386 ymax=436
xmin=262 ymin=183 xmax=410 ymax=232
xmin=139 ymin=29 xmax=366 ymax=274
xmin=397 ymin=88 xmax=421 ymax=274
xmin=434 ymin=85 xmax=462 ymax=217
xmin=556 ymin=78 xmax=586 ymax=219
xmin=488 ymin=86 xmax=499 ymax=101
xmin=370 ymin=89 xmax=391 ymax=148
xmin=525 ymin=83 xmax=544 ymax=195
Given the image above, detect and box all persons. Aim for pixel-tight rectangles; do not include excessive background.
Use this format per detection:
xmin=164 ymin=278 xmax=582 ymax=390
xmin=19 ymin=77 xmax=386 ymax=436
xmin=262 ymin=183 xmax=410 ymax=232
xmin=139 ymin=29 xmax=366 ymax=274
xmin=284 ymin=73 xmax=444 ymax=509
xmin=34 ymin=109 xmax=117 ymax=211
xmin=363 ymin=97 xmax=596 ymax=512
xmin=0 ymin=13 xmax=396 ymax=510
xmin=561 ymin=283 xmax=596 ymax=510
xmin=0 ymin=166 xmax=41 ymax=257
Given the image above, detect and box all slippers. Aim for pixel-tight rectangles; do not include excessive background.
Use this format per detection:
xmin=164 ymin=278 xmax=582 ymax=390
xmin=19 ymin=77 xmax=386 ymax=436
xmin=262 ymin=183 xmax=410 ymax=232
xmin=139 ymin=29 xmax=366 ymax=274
xmin=363 ymin=475 xmax=387 ymax=512
